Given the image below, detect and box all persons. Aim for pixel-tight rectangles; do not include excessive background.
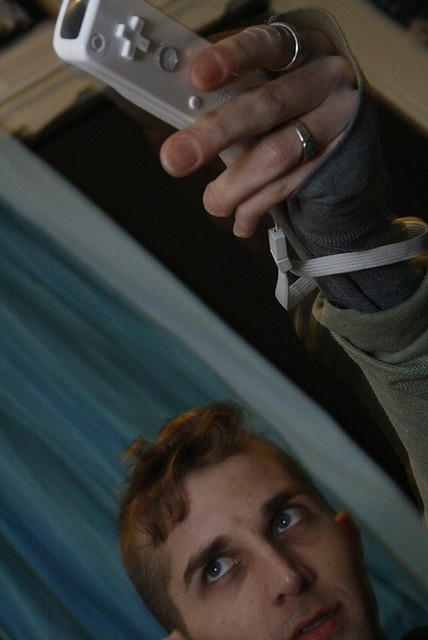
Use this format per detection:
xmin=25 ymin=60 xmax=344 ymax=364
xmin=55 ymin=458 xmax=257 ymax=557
xmin=116 ymin=6 xmax=425 ymax=638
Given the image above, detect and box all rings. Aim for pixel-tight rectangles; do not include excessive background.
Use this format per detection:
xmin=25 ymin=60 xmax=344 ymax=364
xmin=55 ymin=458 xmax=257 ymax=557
xmin=290 ymin=119 xmax=317 ymax=164
xmin=269 ymin=20 xmax=305 ymax=73
